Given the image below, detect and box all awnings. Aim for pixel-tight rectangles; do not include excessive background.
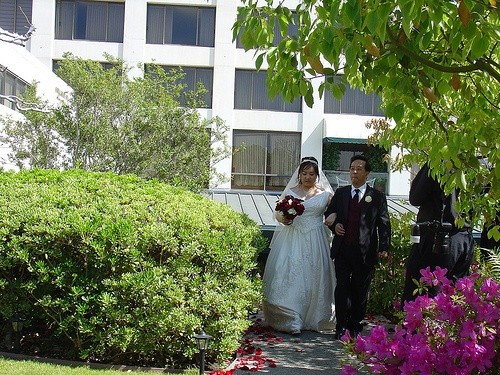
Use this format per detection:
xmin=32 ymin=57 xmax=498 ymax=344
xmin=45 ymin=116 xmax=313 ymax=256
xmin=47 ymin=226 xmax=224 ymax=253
xmin=323 ymin=137 xmax=381 ymax=144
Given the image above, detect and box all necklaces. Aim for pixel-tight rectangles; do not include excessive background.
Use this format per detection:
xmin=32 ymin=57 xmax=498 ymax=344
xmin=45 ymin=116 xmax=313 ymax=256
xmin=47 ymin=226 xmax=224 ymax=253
xmin=302 ymin=186 xmax=312 ymax=193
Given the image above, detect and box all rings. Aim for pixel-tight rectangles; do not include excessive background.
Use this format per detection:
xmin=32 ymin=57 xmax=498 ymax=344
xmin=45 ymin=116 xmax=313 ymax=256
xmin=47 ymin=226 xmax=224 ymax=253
xmin=328 ymin=224 xmax=329 ymax=225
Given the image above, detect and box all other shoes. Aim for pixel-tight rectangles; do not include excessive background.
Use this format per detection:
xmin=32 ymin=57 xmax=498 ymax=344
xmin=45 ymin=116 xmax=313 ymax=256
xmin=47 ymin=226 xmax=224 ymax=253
xmin=292 ymin=330 xmax=301 ymax=334
xmin=335 ymin=326 xmax=346 ymax=339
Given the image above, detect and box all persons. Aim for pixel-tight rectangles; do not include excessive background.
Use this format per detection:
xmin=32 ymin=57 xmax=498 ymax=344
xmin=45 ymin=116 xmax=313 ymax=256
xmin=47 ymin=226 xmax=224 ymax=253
xmin=400 ymin=161 xmax=491 ymax=313
xmin=261 ymin=157 xmax=336 ymax=335
xmin=323 ymin=155 xmax=391 ymax=340
xmin=480 ymin=184 xmax=500 ymax=268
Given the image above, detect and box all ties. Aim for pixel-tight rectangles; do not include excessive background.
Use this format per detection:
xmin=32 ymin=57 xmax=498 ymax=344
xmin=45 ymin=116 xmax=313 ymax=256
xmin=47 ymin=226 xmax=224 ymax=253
xmin=353 ymin=189 xmax=360 ymax=207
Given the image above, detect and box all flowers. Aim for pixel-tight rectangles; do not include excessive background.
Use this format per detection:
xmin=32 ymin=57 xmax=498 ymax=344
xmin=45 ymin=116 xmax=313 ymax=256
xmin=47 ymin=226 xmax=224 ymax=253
xmin=276 ymin=196 xmax=305 ymax=226
xmin=365 ymin=195 xmax=372 ymax=202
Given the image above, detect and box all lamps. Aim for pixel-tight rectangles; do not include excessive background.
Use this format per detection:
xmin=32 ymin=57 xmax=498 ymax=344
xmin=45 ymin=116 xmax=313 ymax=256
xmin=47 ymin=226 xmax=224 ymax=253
xmin=191 ymin=329 xmax=214 ymax=351
